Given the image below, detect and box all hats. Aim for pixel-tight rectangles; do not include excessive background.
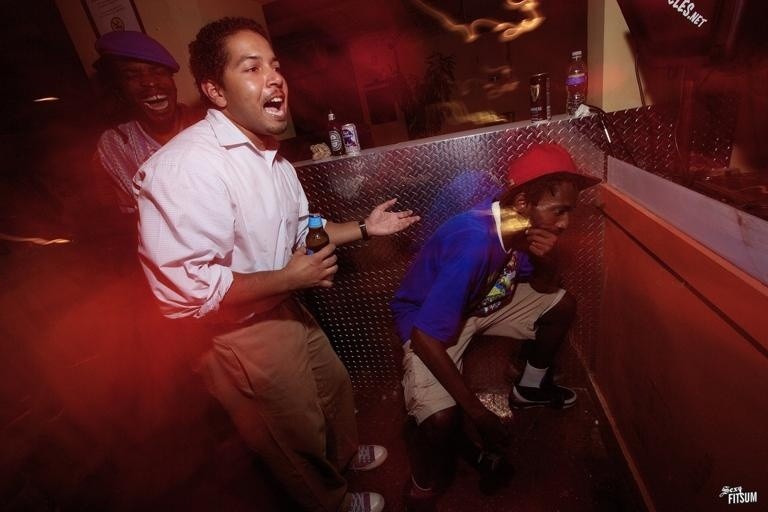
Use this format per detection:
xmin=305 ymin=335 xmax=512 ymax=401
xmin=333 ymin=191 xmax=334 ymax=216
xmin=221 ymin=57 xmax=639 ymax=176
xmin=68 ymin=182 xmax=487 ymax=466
xmin=502 ymin=140 xmax=603 ymax=195
xmin=94 ymin=29 xmax=183 ymax=75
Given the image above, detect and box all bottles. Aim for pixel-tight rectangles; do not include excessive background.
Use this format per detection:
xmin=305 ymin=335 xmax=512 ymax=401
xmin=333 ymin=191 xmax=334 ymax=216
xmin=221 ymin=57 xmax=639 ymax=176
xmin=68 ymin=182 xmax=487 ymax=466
xmin=305 ymin=216 xmax=332 ymax=281
xmin=566 ymin=50 xmax=588 ymax=112
xmin=325 ymin=107 xmax=344 ymax=157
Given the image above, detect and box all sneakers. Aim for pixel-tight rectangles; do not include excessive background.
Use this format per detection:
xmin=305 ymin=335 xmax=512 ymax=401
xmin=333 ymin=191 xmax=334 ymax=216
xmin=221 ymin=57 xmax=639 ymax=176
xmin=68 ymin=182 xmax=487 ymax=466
xmin=344 ymin=491 xmax=386 ymax=512
xmin=337 ymin=442 xmax=389 ymax=473
xmin=409 ymin=448 xmax=437 ymax=493
xmin=508 ymin=374 xmax=579 ymax=412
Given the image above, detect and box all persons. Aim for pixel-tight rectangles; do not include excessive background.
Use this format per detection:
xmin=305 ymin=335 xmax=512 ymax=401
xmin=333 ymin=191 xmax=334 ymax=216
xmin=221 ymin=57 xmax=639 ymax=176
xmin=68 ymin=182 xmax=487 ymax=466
xmin=390 ymin=144 xmax=603 ymax=493
xmin=85 ymin=28 xmax=212 ymax=241
xmin=131 ymin=16 xmax=422 ymax=512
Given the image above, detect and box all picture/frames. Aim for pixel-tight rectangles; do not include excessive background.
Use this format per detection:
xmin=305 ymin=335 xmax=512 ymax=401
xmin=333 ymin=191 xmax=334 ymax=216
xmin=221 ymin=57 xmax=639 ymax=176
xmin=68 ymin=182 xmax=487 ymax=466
xmin=80 ymin=1 xmax=149 ymax=39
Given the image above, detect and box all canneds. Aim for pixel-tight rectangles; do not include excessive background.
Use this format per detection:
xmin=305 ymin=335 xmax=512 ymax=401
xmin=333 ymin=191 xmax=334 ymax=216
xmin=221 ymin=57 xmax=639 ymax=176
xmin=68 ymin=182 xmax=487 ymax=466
xmin=341 ymin=124 xmax=360 ymax=154
xmin=529 ymin=72 xmax=552 ymax=124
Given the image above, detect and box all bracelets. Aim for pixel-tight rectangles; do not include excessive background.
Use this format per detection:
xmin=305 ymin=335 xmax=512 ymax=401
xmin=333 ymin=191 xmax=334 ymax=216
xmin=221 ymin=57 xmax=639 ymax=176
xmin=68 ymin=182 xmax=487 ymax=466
xmin=358 ymin=220 xmax=370 ymax=243
